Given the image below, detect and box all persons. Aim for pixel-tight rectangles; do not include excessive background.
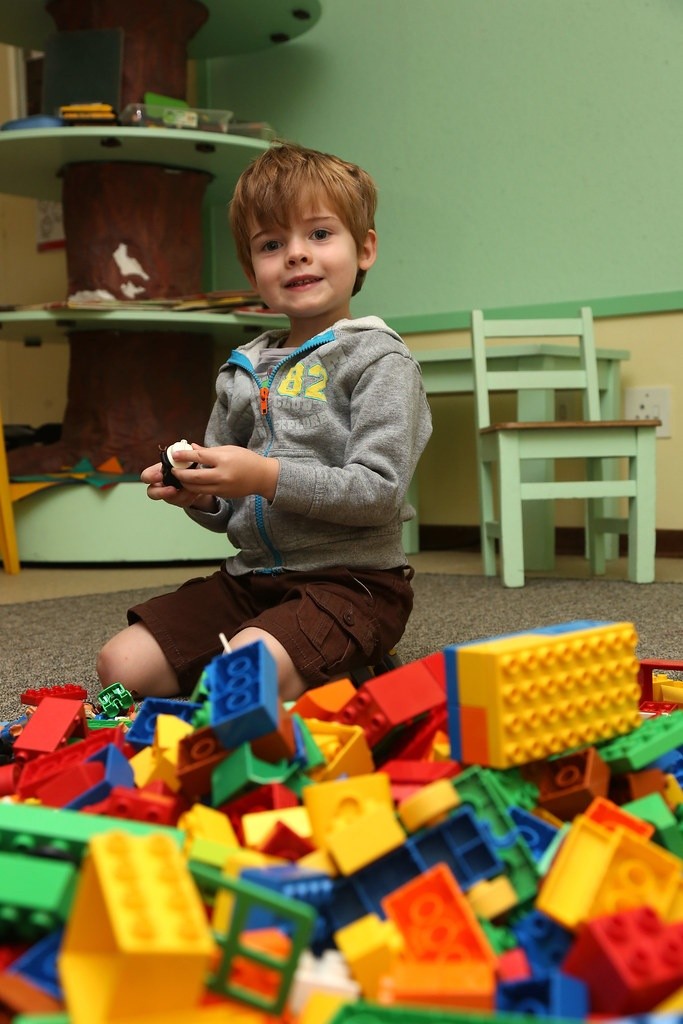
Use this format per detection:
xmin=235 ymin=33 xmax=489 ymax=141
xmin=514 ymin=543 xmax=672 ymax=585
xmin=95 ymin=140 xmax=436 ymax=726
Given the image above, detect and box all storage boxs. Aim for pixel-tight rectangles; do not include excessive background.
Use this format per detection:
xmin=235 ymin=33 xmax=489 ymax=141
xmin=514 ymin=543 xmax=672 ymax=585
xmin=119 ymin=102 xmax=233 ymax=134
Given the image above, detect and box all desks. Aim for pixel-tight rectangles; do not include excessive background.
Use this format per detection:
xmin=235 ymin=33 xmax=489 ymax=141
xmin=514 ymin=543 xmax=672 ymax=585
xmin=402 ymin=342 xmax=630 ymax=570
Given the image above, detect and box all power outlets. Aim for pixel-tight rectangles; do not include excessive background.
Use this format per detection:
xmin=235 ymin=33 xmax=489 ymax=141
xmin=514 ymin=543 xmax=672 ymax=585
xmin=625 ymin=385 xmax=672 ymax=437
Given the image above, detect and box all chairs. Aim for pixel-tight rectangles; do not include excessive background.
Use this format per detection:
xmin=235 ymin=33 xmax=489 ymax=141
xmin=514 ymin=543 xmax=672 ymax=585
xmin=470 ymin=307 xmax=663 ymax=588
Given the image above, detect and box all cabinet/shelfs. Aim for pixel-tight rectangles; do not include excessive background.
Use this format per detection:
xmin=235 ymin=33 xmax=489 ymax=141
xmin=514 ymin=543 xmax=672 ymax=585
xmin=0 ymin=0 xmax=323 ymax=562
